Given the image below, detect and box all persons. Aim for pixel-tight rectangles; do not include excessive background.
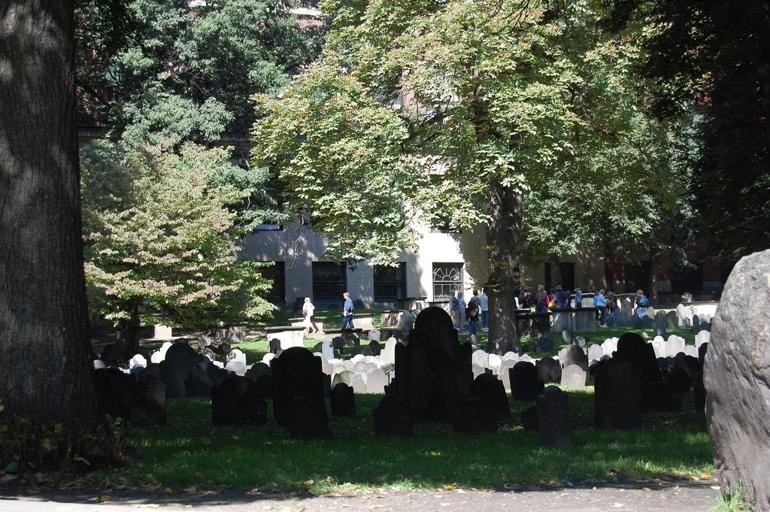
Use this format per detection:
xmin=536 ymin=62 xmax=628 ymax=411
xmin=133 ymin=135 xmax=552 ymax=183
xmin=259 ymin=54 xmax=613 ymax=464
xmin=339 ymin=292 xmax=354 ymax=330
xmin=635 ymin=289 xmax=650 ymax=307
xmin=447 ymin=286 xmax=490 ymax=334
xmin=466 ymin=302 xmax=479 ymax=345
xmin=303 ymin=297 xmax=317 ymax=333
xmin=518 ymin=280 xmax=616 ymax=332
